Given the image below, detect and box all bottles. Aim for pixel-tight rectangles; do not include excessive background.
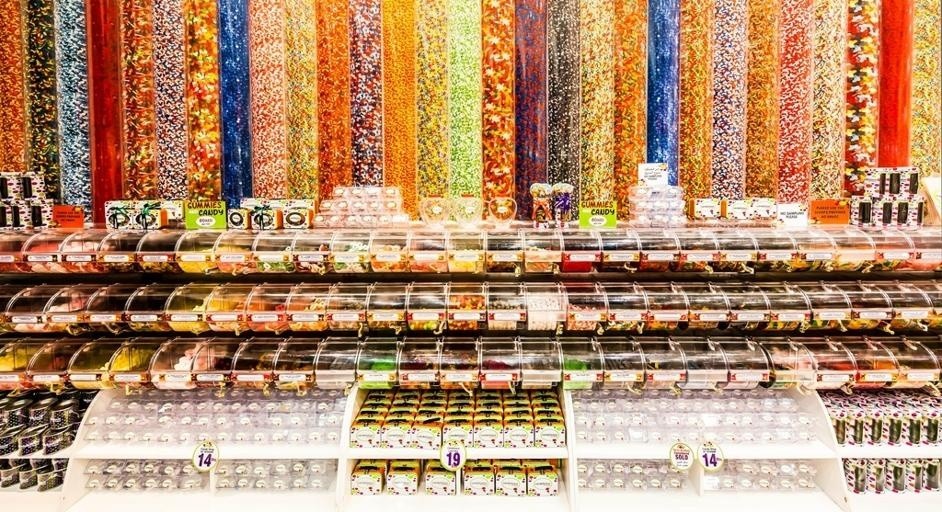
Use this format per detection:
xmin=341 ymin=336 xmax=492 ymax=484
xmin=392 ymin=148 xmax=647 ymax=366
xmin=416 ymin=192 xmax=517 ymax=230
